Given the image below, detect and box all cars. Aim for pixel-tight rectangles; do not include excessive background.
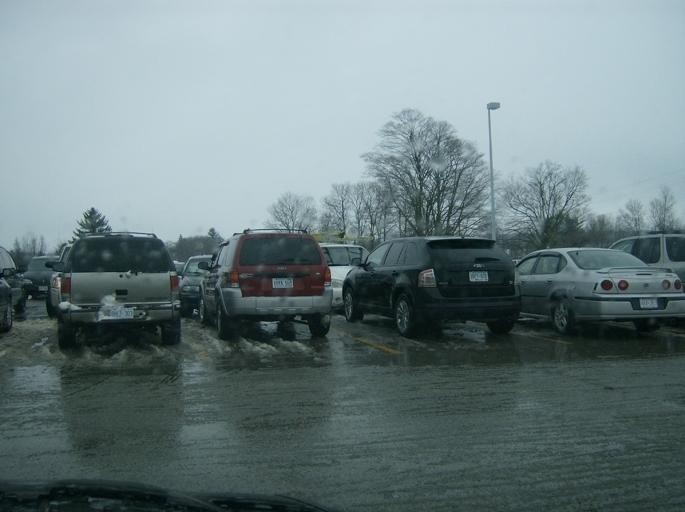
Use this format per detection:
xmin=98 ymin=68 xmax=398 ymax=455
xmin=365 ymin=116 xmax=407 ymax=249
xmin=609 ymin=234 xmax=685 ymax=326
xmin=513 ymin=245 xmax=685 ymax=338
xmin=50 ymin=231 xmax=180 ymax=349
xmin=313 ymin=241 xmax=367 ymax=311
xmin=196 ymin=229 xmax=334 ymax=337
xmin=21 ymin=255 xmax=60 ymax=302
xmin=340 ymin=234 xmax=521 ymax=336
xmin=45 ymin=243 xmax=73 ymax=317
xmin=0 ymin=246 xmax=32 ymax=333
xmin=175 ymin=253 xmax=220 ymax=318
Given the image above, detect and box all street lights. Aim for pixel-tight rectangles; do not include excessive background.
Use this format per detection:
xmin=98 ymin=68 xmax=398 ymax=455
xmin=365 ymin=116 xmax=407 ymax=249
xmin=484 ymin=101 xmax=502 ymax=244
